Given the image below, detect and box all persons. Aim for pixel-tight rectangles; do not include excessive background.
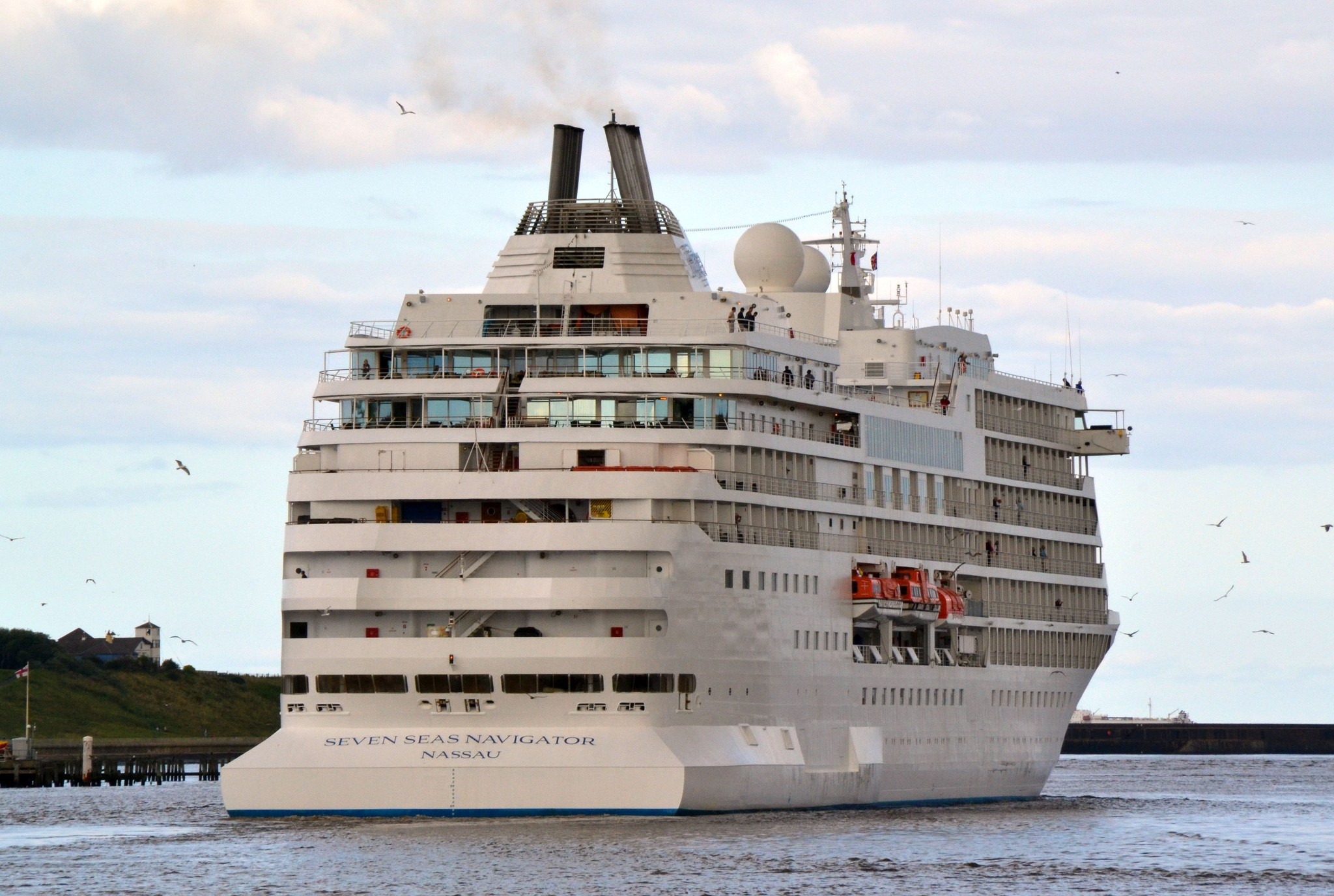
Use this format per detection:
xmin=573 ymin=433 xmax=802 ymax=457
xmin=737 ymin=307 xmax=758 ymax=332
xmin=940 ymin=395 xmax=950 ymax=415
xmin=1022 ymin=455 xmax=1031 ymax=478
xmin=804 ymin=370 xmax=815 ymax=389
xmin=1062 ymin=378 xmax=1071 ymax=388
xmin=1076 ymin=381 xmax=1084 ymax=396
xmin=1012 ymin=499 xmax=1024 ymax=526
xmin=517 ymin=370 xmax=525 ymax=381
xmin=994 ymin=540 xmax=1000 ymax=555
xmin=754 ymin=367 xmax=764 ymax=380
xmin=958 ymin=351 xmax=971 ymax=375
xmin=993 ymin=497 xmax=1002 ymax=521
xmin=782 ymin=366 xmax=794 ymax=385
xmin=727 ymin=307 xmax=736 ymax=333
xmin=361 ymin=359 xmax=371 ymax=380
xmin=1055 ymin=598 xmax=1063 ymax=621
xmin=764 ymin=369 xmax=771 ymax=381
xmin=665 ymin=369 xmax=671 ymax=377
xmin=986 ymin=539 xmax=994 ymax=566
xmin=432 ymin=365 xmax=439 ymax=373
xmin=1027 ymin=546 xmax=1037 ymax=568
xmin=1040 ymin=545 xmax=1048 ymax=571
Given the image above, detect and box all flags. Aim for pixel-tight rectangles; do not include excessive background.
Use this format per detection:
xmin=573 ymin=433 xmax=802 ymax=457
xmin=15 ymin=664 xmax=29 ymax=678
xmin=871 ymin=252 xmax=877 ymax=271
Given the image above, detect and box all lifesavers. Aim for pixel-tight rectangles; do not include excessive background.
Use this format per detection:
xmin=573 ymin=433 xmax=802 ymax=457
xmin=961 ymin=361 xmax=967 ymax=372
xmin=397 ymin=326 xmax=410 ymax=339
xmin=845 ymin=436 xmax=848 ymax=446
xmin=471 ymin=368 xmax=484 ymax=377
xmin=773 ymin=424 xmax=780 ymax=432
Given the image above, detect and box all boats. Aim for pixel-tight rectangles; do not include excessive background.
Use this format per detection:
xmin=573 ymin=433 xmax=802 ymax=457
xmin=849 ymin=567 xmax=905 ymax=620
xmin=898 ymin=572 xmax=941 ymax=626
xmin=218 ymin=120 xmax=1137 ymax=817
xmin=937 ymin=587 xmax=966 ymax=627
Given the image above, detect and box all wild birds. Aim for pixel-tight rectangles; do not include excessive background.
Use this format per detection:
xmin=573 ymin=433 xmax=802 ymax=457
xmin=1119 ymin=629 xmax=1140 ymax=637
xmin=1251 ymin=629 xmax=1275 ymax=635
xmin=944 ymin=533 xmax=958 ymax=544
xmin=174 ymin=459 xmax=191 ymax=476
xmin=0 ymin=534 xmax=26 ymax=542
xmin=1213 ymin=584 xmax=1234 ymax=601
xmin=778 ymin=524 xmax=803 ymax=533
xmin=40 ymin=602 xmax=47 ymax=606
xmin=169 ymin=635 xmax=198 ymax=647
xmin=1204 ymin=516 xmax=1228 ymax=527
xmin=519 ymin=687 xmax=548 ymax=700
xmin=1319 ymin=523 xmax=1334 ymax=532
xmin=1115 ymin=71 xmax=1120 ymax=74
xmin=1048 ymin=671 xmax=1067 ymax=677
xmin=964 ymin=551 xmax=983 ymax=558
xmin=1120 ymin=592 xmax=1138 ymax=601
xmin=315 ymin=605 xmax=331 ymax=617
xmin=1234 ymin=221 xmax=1256 ymax=225
xmin=1106 ymin=373 xmax=1127 ymax=377
xmin=417 ymin=289 xmax=425 ymax=294
xmin=1001 ymin=505 xmax=1017 ymax=509
xmin=1010 ymin=404 xmax=1028 ymax=411
xmin=396 ymin=100 xmax=416 ymax=116
xmin=1240 ymin=550 xmax=1250 ymax=564
xmin=86 ymin=579 xmax=96 ymax=584
